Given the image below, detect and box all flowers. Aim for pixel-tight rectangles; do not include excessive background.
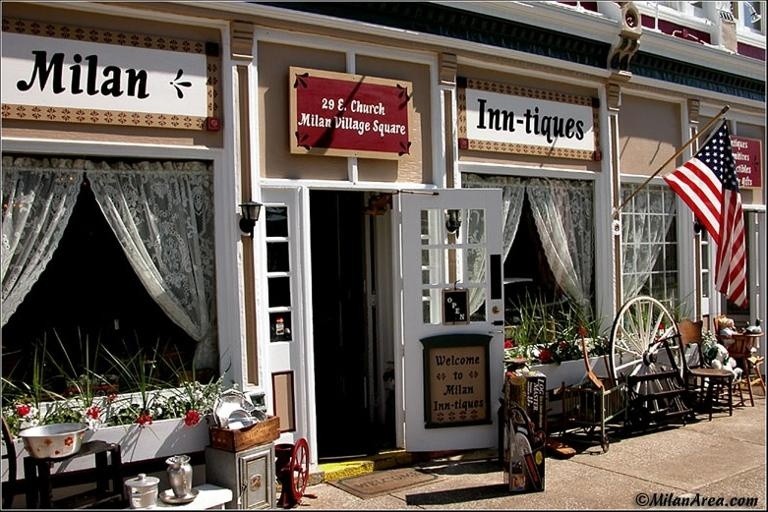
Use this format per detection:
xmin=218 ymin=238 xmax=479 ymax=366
xmin=504 ymin=282 xmax=711 ymax=370
xmin=1 ymin=325 xmax=246 ymax=440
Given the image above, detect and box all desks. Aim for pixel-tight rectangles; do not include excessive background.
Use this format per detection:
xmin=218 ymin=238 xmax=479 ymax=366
xmin=125 ymin=484 xmax=233 ymax=510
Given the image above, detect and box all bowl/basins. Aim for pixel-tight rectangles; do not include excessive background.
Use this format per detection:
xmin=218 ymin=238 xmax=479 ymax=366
xmin=17 ymin=423 xmax=89 ymax=459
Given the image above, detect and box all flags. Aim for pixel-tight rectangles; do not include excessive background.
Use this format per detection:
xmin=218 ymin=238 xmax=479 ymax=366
xmin=657 ymin=103 xmax=749 ymax=310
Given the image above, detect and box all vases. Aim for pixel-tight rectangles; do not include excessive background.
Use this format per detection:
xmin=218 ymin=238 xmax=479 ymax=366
xmin=504 ymin=331 xmax=675 ymax=414
xmin=1 ymin=383 xmax=219 ymax=482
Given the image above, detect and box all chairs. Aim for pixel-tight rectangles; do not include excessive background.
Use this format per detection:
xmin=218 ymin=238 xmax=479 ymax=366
xmin=703 ymin=352 xmax=747 ymax=410
xmin=728 ymin=352 xmax=765 ymax=407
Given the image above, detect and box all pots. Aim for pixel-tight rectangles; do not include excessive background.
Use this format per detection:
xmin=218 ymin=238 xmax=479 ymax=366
xmin=124 ymin=473 xmax=161 ymax=509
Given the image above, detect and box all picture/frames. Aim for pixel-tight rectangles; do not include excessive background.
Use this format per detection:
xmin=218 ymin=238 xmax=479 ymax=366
xmin=441 ymin=288 xmax=470 ymax=325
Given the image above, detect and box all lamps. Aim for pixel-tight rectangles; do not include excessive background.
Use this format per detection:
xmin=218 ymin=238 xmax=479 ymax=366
xmin=238 ymin=200 xmax=263 ymax=237
xmin=445 ymin=208 xmax=462 ymax=235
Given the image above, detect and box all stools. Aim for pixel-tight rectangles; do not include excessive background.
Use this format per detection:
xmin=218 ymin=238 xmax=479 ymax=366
xmin=686 ymin=368 xmax=734 ymax=422
xmin=23 ymin=437 xmax=130 ymax=510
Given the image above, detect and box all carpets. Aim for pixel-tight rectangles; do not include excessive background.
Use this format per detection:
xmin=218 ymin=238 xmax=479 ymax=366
xmin=325 ymin=466 xmax=449 ymax=501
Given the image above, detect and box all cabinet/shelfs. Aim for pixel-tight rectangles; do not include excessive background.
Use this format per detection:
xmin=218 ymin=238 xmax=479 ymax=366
xmin=205 ymin=441 xmax=277 ymax=510
